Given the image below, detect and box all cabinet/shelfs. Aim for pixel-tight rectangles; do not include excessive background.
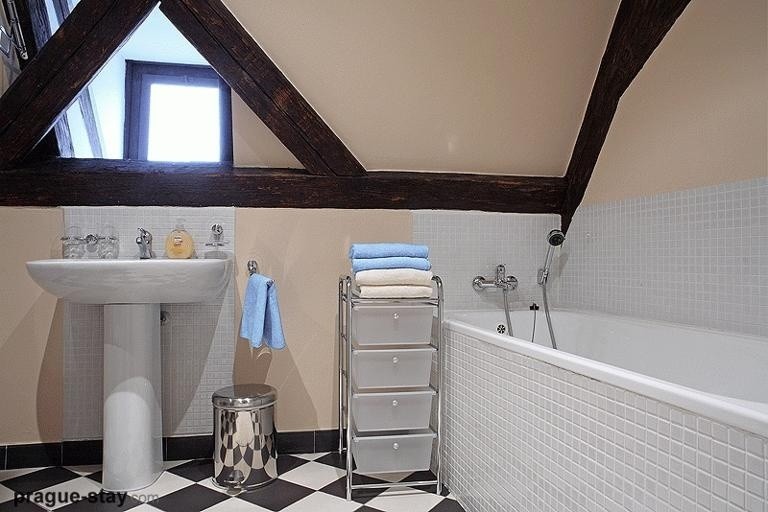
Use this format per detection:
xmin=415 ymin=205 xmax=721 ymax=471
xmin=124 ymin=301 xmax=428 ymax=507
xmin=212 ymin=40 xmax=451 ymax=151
xmin=337 ymin=275 xmax=445 ymax=500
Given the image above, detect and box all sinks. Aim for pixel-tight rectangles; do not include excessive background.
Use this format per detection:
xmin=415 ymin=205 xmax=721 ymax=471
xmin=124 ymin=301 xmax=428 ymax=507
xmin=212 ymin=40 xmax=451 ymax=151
xmin=24 ymin=256 xmax=229 ymax=305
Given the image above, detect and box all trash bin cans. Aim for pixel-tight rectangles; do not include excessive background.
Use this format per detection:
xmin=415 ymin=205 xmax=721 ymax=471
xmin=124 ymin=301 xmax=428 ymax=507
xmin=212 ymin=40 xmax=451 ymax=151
xmin=211 ymin=384 xmax=279 ymax=497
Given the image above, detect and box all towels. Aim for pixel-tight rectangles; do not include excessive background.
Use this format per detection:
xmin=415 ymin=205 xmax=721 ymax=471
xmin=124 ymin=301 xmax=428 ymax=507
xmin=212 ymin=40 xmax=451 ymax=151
xmin=350 ymin=243 xmax=433 ymax=298
xmin=239 ymin=274 xmax=286 ymax=350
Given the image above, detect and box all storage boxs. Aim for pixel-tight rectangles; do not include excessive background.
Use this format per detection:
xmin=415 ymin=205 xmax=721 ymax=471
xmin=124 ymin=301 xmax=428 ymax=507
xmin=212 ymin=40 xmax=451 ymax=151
xmin=348 ymin=302 xmax=436 ymax=474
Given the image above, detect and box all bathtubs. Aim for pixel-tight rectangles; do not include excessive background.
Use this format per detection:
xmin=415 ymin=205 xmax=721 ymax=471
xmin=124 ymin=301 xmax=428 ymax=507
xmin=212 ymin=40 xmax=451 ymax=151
xmin=431 ymin=305 xmax=768 ymax=440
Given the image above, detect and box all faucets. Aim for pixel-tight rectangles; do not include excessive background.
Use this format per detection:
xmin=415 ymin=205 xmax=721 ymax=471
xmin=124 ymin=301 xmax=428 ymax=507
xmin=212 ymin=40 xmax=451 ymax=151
xmin=473 ymin=264 xmax=519 ymax=293
xmin=135 ymin=227 xmax=154 ymax=258
